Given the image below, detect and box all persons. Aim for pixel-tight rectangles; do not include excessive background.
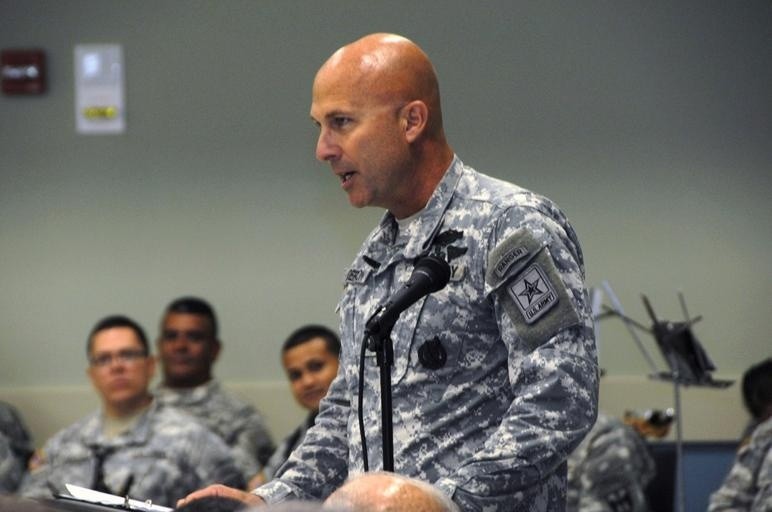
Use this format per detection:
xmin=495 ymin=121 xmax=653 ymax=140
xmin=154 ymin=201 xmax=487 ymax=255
xmin=318 ymin=474 xmax=452 ymax=512
xmin=708 ymin=415 xmax=770 ymax=512
xmin=17 ymin=314 xmax=266 ymax=510
xmin=566 ymin=414 xmax=656 ymax=511
xmin=246 ymin=323 xmax=343 ymax=492
xmin=1 ymin=402 xmax=36 ymax=495
xmin=155 ymin=298 xmax=277 ymax=469
xmin=175 ymin=31 xmax=601 ymax=511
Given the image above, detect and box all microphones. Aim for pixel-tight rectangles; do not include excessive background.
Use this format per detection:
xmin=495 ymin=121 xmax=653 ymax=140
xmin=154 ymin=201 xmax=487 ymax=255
xmin=365 ymin=256 xmax=450 ymax=333
